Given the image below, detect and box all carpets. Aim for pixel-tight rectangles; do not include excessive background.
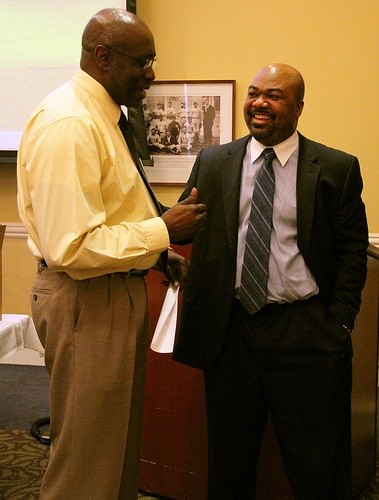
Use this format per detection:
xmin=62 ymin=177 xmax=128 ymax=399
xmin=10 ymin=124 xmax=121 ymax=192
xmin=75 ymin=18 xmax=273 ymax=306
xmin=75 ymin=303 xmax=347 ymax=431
xmin=0 ymin=430 xmax=156 ymax=500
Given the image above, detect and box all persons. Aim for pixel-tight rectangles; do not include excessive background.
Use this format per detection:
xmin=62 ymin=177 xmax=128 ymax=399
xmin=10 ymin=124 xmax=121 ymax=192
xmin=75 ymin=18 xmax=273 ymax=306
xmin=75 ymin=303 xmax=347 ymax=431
xmin=140 ymin=97 xmax=216 ymax=156
xmin=155 ymin=63 xmax=370 ymax=500
xmin=16 ymin=9 xmax=209 ymax=500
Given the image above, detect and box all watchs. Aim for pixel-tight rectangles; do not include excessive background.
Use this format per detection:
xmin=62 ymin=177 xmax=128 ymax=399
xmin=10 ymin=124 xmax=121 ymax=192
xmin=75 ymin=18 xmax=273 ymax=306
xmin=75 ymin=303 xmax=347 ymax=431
xmin=344 ymin=325 xmax=352 ymax=334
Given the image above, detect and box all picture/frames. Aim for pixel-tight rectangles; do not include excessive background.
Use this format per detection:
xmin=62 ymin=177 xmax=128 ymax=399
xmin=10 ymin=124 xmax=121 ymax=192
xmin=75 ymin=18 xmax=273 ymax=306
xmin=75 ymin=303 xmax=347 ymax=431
xmin=143 ymin=80 xmax=237 ymax=186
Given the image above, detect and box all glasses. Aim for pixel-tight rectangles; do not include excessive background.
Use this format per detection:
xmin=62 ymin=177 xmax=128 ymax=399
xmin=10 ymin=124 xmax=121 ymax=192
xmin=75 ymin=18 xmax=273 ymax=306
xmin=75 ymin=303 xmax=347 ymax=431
xmin=103 ymin=45 xmax=156 ymax=70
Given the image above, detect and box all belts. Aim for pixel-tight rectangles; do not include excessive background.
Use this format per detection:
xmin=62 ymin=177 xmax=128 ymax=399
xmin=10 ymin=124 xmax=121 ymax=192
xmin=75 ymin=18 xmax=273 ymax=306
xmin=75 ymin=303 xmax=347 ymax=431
xmin=40 ymin=259 xmax=149 ymax=278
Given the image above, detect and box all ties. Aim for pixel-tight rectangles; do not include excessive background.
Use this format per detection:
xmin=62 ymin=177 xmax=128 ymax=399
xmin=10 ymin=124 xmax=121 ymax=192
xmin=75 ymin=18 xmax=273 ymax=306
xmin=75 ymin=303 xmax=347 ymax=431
xmin=118 ymin=110 xmax=168 ymax=272
xmin=238 ymin=148 xmax=278 ymax=316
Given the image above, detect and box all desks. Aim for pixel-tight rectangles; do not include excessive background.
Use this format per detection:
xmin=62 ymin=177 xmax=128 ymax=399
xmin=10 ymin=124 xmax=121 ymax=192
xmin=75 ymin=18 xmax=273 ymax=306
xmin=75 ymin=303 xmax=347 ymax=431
xmin=0 ymin=314 xmax=45 ymax=367
